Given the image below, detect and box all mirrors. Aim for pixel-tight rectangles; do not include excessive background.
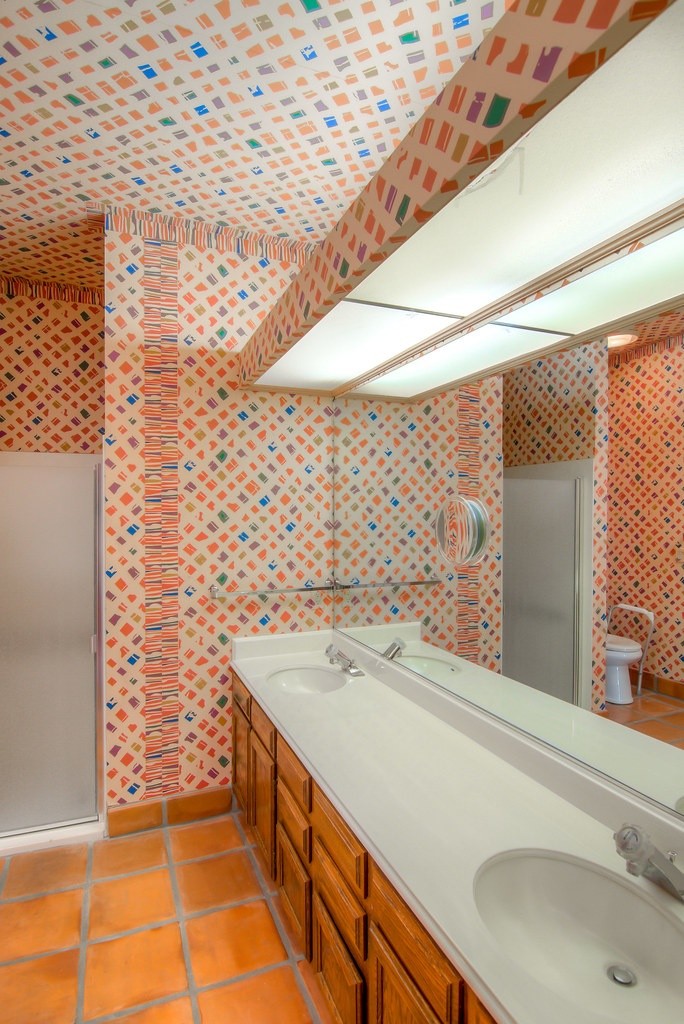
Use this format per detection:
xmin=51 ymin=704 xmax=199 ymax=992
xmin=331 ymin=214 xmax=683 ymax=821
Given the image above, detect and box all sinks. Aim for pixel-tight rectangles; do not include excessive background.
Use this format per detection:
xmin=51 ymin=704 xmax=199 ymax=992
xmin=385 ymin=650 xmax=476 ymax=692
xmin=471 ymin=844 xmax=684 ymax=1023
xmin=264 ymin=664 xmax=351 ymax=696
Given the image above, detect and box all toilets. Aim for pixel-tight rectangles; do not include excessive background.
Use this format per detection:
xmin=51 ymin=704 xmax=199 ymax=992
xmin=605 ymin=629 xmax=643 ymax=705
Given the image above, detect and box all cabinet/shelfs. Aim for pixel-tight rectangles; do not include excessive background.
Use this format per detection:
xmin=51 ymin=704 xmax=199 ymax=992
xmin=231 ymin=669 xmax=495 ymax=1024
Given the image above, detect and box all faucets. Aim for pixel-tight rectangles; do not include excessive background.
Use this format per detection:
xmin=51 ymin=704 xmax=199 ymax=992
xmin=615 ymin=823 xmax=683 ymax=899
xmin=324 ymin=644 xmax=365 ymax=677
xmin=381 ymin=637 xmax=407 ymax=664
